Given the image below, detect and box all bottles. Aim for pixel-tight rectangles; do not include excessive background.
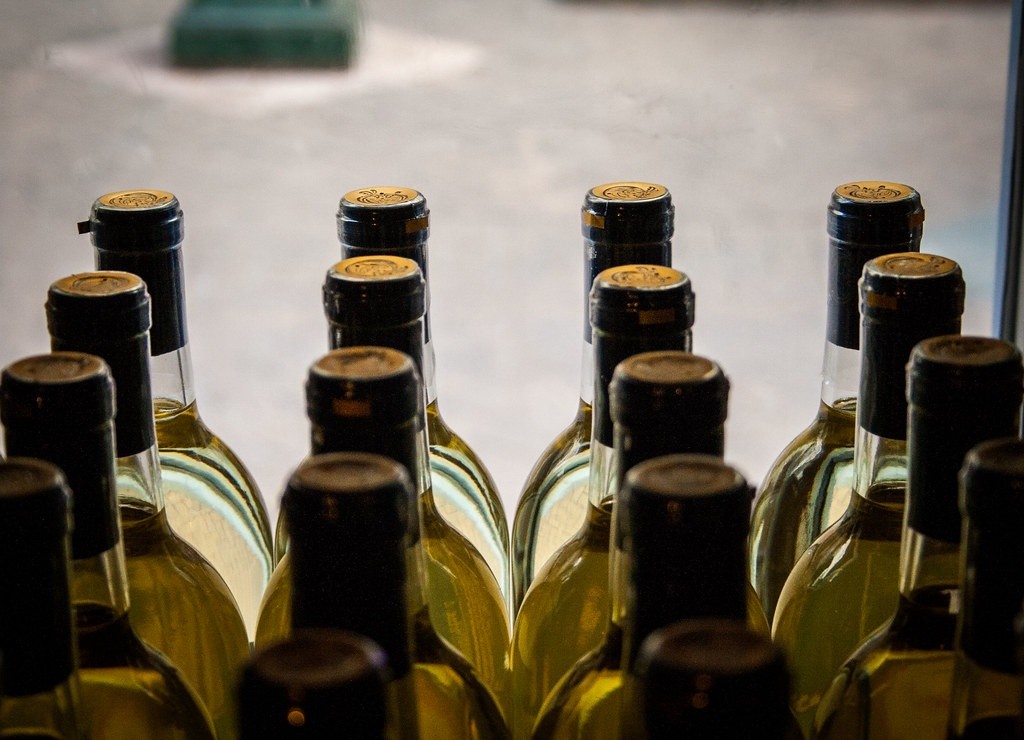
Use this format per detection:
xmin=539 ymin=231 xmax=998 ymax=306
xmin=43 ymin=273 xmax=250 ymax=740
xmin=83 ymin=189 xmax=272 ymax=649
xmin=254 ymin=185 xmax=1022 ymax=740
xmin=3 ymin=350 xmax=217 ymax=737
xmin=0 ymin=458 xmax=91 ymax=739
